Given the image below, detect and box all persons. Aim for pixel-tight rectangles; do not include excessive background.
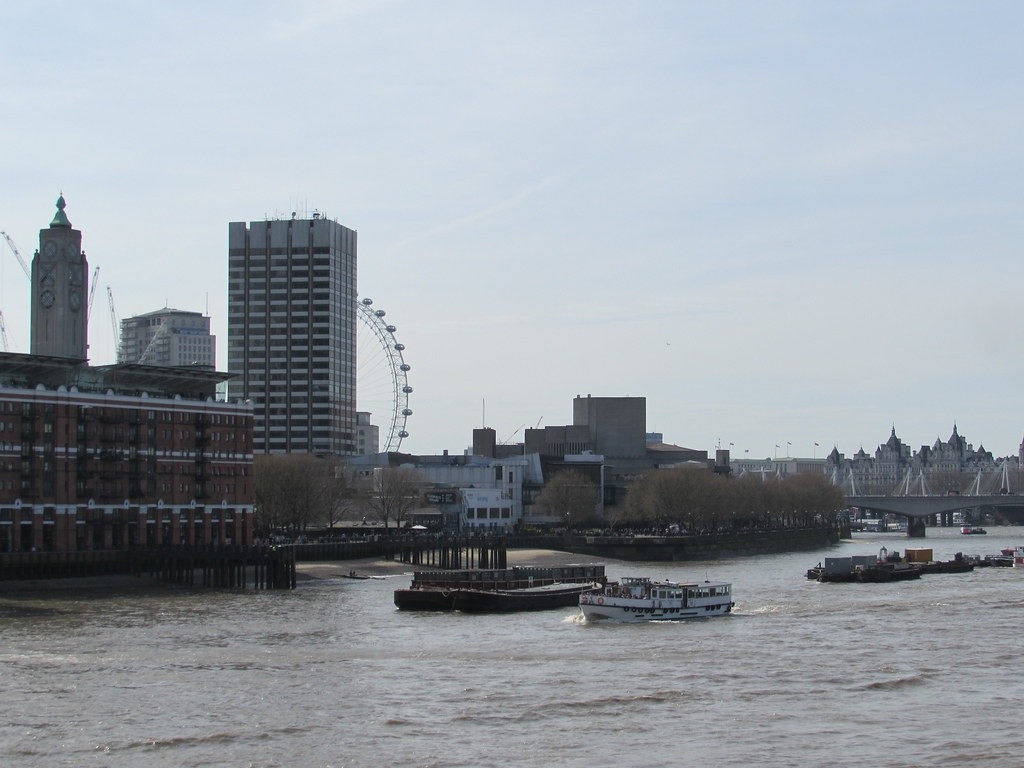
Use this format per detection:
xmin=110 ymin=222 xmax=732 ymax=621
xmin=349 ymin=569 xmax=356 ymax=576
xmin=587 ymin=591 xmax=649 ymax=601
xmin=265 ymin=525 xmax=748 ymax=544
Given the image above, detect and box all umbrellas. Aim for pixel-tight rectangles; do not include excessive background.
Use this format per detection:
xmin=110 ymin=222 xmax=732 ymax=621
xmin=412 ymin=525 xmax=428 ymax=534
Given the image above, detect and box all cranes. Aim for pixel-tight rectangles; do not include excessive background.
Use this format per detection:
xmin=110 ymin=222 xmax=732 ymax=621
xmin=0 ymin=230 xmax=31 ymax=281
xmin=0 ymin=311 xmax=11 ymax=352
xmin=87 ymin=265 xmax=100 ymax=322
xmin=106 ymin=285 xmax=120 ymax=351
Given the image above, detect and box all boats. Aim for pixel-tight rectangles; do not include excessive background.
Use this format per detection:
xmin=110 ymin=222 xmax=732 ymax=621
xmin=394 ymin=564 xmax=608 ymax=612
xmin=576 ymin=576 xmax=735 ymax=622
xmin=806 ymin=545 xmax=1024 ymax=583
xmin=959 ymin=523 xmax=987 ymax=534
